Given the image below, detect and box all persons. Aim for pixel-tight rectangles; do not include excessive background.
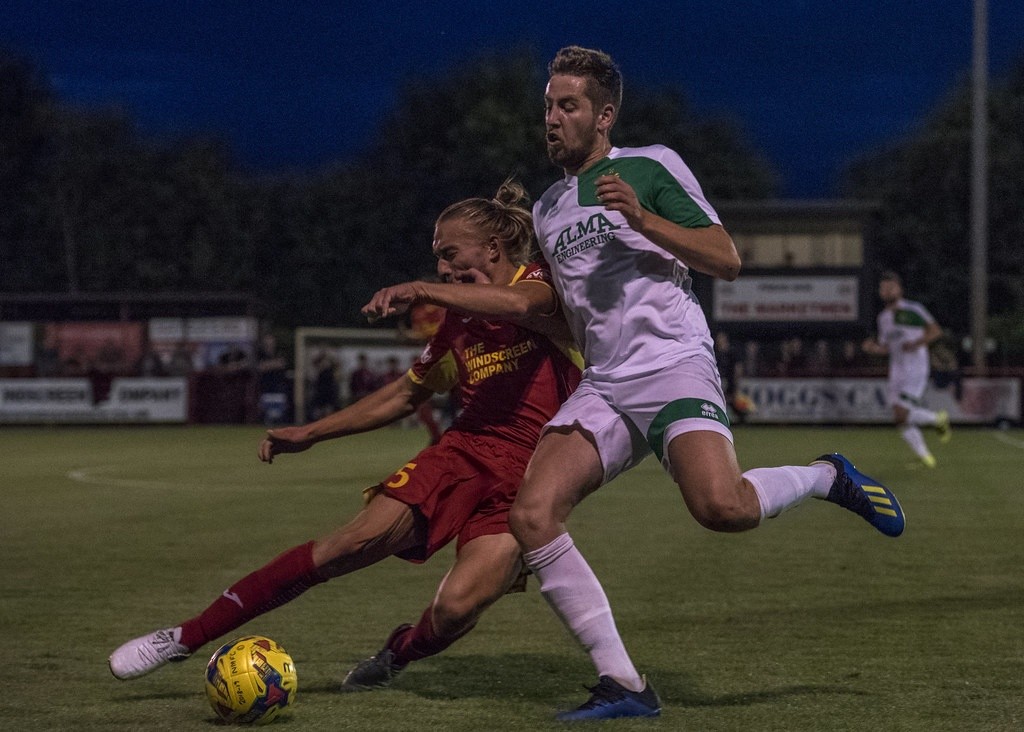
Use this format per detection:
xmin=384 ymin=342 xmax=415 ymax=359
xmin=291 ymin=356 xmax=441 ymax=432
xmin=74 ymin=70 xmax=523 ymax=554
xmin=508 ymin=44 xmax=905 ymax=720
xmin=860 ymin=268 xmax=951 ymax=471
xmin=1 ymin=300 xmax=960 ymax=447
xmin=108 ymin=186 xmax=587 ymax=697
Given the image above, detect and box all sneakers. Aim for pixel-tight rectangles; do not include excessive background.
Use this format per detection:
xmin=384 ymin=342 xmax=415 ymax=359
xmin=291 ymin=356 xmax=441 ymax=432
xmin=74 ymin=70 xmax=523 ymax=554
xmin=340 ymin=623 xmax=417 ymax=693
xmin=554 ymin=674 xmax=662 ymax=722
xmin=108 ymin=626 xmax=192 ymax=680
xmin=808 ymin=452 xmax=906 ymax=537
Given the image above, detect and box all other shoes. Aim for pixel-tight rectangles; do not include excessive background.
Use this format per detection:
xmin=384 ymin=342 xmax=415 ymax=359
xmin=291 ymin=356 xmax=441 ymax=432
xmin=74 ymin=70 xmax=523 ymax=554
xmin=937 ymin=410 xmax=952 ymax=444
xmin=906 ymin=455 xmax=938 ymax=472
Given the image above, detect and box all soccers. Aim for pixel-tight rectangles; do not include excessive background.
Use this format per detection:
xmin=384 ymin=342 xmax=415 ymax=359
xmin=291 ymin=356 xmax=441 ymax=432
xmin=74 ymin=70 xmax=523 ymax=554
xmin=203 ymin=634 xmax=299 ymax=726
